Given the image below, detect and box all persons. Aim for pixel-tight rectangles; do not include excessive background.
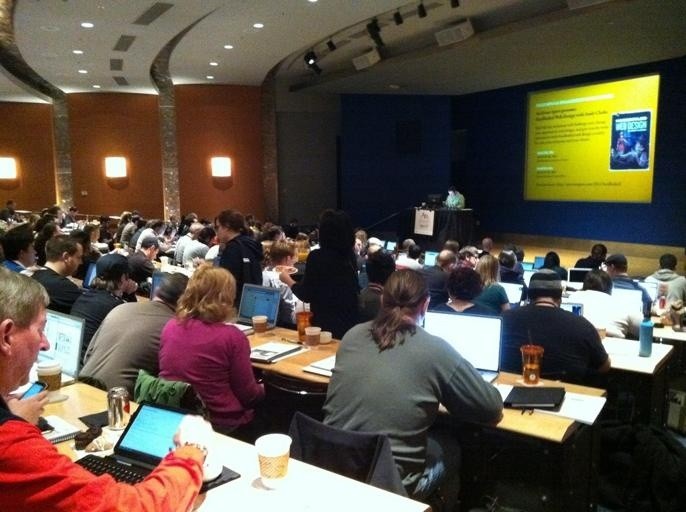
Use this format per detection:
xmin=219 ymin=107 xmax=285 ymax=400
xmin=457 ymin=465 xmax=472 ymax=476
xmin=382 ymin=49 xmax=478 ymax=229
xmin=610 ymin=131 xmax=648 ymax=168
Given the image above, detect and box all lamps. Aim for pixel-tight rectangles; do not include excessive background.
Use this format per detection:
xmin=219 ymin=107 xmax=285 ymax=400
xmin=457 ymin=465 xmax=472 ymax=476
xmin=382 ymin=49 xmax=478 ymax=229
xmin=211 ymin=156 xmax=231 ymax=177
xmin=286 ymin=0 xmax=460 ymax=68
xmin=104 ymin=156 xmax=127 ymax=178
xmin=0 ymin=157 xmax=16 ymax=178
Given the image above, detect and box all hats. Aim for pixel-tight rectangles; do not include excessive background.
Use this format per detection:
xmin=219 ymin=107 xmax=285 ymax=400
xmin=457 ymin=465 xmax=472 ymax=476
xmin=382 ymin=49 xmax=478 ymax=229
xmin=95 ymin=254 xmax=134 ymax=282
xmin=528 ymin=269 xmax=562 ymax=300
xmin=604 ymin=253 xmax=627 ymax=266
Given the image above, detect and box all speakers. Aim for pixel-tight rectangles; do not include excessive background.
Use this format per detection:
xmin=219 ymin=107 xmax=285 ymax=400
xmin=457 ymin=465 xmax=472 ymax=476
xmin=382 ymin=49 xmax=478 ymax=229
xmin=435 ymin=20 xmax=474 ymax=47
xmin=353 ymin=49 xmax=381 ymax=70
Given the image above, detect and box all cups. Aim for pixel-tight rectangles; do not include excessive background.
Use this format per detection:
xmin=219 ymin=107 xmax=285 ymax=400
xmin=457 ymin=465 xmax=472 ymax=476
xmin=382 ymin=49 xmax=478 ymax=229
xmin=36 ymin=360 xmax=61 ymax=393
xmin=519 ymin=344 xmax=544 ymax=386
xmin=160 ymin=256 xmax=168 ymax=268
xmin=253 ymin=432 xmax=292 ymax=490
xmin=318 ymin=331 xmax=332 ymax=345
xmin=295 ymin=312 xmax=312 ymax=341
xmin=304 ymin=326 xmax=321 ymax=345
xmin=251 ymin=315 xmax=267 ymax=337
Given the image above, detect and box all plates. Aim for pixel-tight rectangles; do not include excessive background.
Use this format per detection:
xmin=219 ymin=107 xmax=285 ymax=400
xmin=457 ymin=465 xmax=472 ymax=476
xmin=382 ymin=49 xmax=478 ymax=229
xmin=272 ymin=268 xmax=298 ymax=275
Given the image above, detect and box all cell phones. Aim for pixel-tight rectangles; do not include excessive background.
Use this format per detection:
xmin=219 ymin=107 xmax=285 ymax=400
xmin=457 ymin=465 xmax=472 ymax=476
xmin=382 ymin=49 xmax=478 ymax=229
xmin=19 ymin=381 xmax=48 ymax=400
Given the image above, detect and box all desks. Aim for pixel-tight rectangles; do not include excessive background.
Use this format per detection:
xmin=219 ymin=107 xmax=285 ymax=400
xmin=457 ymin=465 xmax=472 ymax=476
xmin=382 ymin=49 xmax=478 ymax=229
xmin=1 ymin=219 xmax=686 ymax=511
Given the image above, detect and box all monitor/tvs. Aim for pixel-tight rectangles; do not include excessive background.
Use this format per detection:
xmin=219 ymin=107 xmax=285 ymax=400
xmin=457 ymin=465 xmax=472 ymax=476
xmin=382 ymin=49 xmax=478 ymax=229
xmin=428 ymin=194 xmax=443 ymax=209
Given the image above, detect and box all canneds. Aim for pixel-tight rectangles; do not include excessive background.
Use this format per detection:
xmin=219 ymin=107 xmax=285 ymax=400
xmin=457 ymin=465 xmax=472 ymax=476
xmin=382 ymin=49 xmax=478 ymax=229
xmin=107 ymin=387 xmax=130 ymax=431
xmin=572 ymin=306 xmax=582 ymax=315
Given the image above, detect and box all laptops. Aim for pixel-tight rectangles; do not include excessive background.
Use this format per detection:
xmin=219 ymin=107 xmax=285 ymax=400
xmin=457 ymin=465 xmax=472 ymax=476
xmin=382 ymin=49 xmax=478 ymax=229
xmin=151 ymin=272 xmax=172 ymax=299
xmin=75 ymin=400 xmax=205 ymax=485
xmin=83 ymin=261 xmax=97 ymax=289
xmin=8 ymin=308 xmax=86 ymax=396
xmin=226 ymin=283 xmax=281 ymax=336
xmin=386 ymin=241 xmax=660 ymax=383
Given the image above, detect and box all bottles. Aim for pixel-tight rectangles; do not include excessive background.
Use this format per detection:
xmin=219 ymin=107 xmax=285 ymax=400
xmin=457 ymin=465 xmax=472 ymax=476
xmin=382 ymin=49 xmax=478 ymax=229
xmin=638 ymin=311 xmax=655 ymax=358
xmin=671 ymin=303 xmax=686 ymax=333
xmin=658 ymin=295 xmax=666 ymax=309
